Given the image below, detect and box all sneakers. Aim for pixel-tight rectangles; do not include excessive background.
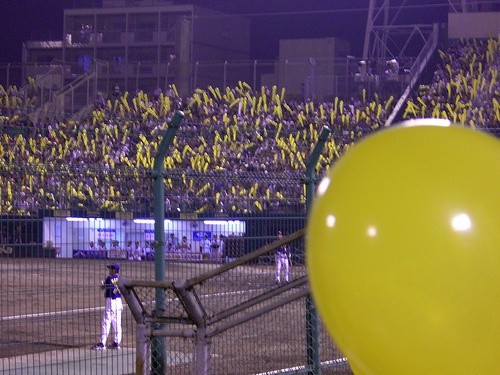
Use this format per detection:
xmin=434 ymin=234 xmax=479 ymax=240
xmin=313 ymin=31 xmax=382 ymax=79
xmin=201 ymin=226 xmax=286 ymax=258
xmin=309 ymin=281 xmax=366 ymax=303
xmin=107 ymin=342 xmax=121 ymax=349
xmin=91 ymin=343 xmax=105 ymax=350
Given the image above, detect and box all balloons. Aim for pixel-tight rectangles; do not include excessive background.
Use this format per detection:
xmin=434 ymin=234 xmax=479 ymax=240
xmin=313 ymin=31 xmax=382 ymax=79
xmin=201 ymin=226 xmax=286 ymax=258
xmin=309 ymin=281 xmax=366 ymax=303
xmin=304 ymin=119 xmax=500 ymax=375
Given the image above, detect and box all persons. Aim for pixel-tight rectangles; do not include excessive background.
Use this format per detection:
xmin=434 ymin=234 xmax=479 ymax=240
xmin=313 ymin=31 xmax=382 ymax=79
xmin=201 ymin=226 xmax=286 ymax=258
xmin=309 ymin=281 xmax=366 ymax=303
xmin=271 ymin=230 xmax=293 ymax=285
xmin=0 ymin=39 xmax=499 ymax=219
xmin=89 ymin=232 xmax=233 ymax=262
xmin=92 ymin=264 xmax=124 ymax=349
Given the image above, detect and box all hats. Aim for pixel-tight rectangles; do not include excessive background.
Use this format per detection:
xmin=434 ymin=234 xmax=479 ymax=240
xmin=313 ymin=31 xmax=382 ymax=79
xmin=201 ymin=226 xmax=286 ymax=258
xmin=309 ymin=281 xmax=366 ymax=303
xmin=277 ymin=231 xmax=282 ymax=235
xmin=106 ymin=264 xmax=119 ymax=272
